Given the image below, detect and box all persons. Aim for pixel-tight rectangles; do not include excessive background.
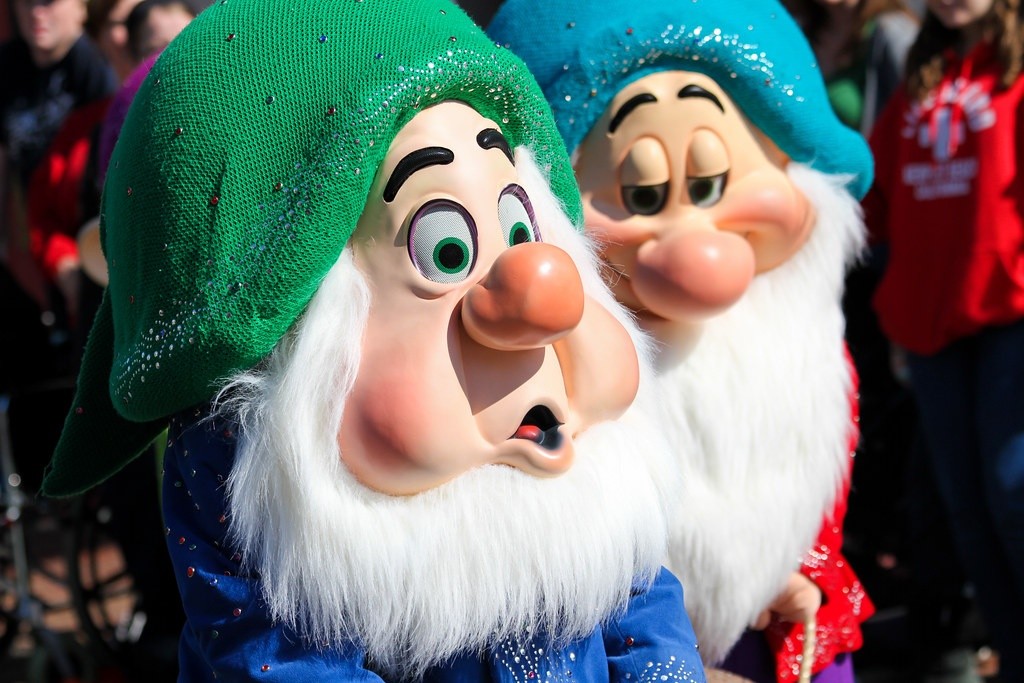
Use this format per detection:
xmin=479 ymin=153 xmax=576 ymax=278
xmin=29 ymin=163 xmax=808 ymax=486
xmin=0 ymin=0 xmax=198 ymax=683
xmin=487 ymin=0 xmax=874 ymax=683
xmin=100 ymin=0 xmax=708 ymax=682
xmin=788 ymin=0 xmax=1024 ymax=682
